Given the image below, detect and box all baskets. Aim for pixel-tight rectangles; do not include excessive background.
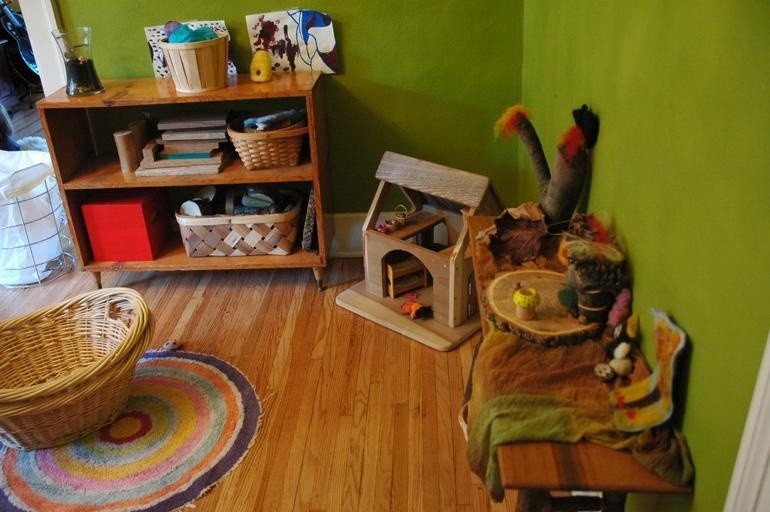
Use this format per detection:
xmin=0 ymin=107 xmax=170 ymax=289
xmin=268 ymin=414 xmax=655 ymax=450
xmin=175 ymin=187 xmax=303 ymax=258
xmin=227 ymin=117 xmax=308 ymax=170
xmin=1 ymin=287 xmax=156 ymax=452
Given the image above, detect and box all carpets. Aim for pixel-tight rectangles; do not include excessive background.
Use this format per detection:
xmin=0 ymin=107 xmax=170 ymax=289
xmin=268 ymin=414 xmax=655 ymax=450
xmin=0 ymin=348 xmax=263 ymax=512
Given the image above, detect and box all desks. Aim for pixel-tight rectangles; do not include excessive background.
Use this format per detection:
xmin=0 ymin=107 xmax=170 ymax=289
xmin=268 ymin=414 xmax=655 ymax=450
xmin=466 ymin=214 xmax=693 ymax=512
xmin=34 ymin=70 xmax=335 ymax=292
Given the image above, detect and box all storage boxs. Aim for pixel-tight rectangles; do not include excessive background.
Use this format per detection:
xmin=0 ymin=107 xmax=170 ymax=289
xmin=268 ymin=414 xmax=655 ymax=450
xmin=81 ymin=193 xmax=168 ymax=261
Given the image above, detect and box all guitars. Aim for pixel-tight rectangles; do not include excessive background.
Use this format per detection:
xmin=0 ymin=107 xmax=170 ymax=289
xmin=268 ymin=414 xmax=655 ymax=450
xmin=0 ymin=0 xmax=40 ymax=84
